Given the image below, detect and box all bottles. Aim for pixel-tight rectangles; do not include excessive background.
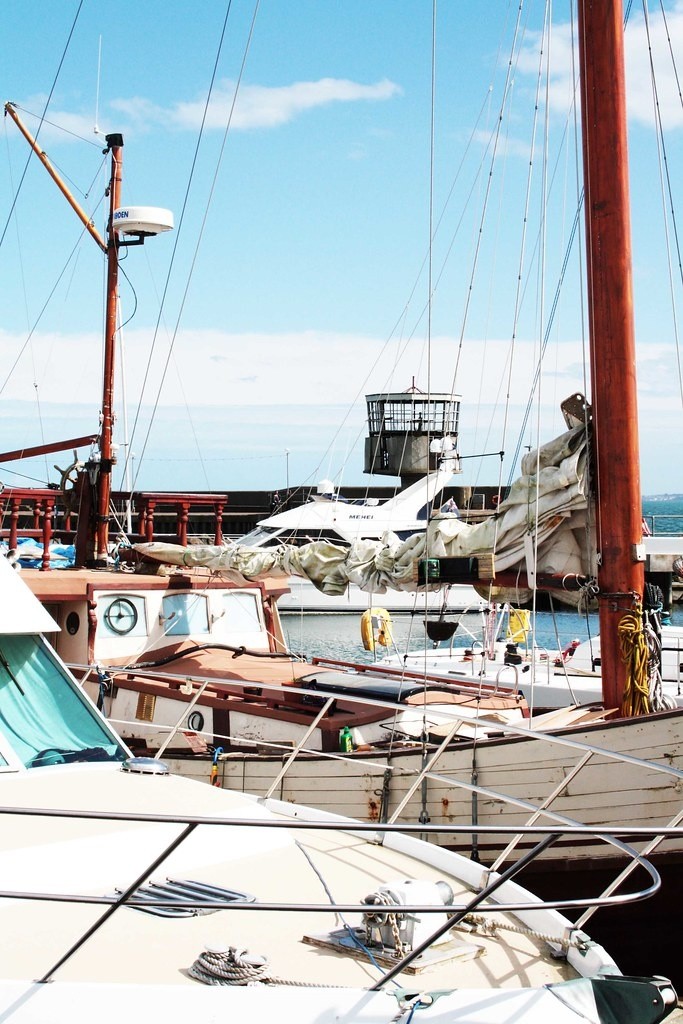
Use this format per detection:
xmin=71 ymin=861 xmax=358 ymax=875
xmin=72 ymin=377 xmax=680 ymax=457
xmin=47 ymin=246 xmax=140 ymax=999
xmin=340 ymin=726 xmax=353 ymax=752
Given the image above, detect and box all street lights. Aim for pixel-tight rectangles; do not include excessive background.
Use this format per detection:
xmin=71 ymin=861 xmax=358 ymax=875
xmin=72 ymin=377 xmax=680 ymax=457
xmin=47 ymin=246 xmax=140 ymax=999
xmin=89 ymin=206 xmax=173 ymax=558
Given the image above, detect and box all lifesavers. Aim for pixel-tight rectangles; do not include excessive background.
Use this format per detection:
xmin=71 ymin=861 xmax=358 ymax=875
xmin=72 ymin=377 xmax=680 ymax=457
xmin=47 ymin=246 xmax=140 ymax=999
xmin=360 ymin=607 xmax=394 ymax=650
xmin=491 ymin=494 xmax=503 ymax=506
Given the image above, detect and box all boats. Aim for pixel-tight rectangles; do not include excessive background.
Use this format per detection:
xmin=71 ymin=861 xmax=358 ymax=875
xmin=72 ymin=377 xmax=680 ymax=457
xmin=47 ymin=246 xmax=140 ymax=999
xmin=0 ymin=0 xmax=683 ymax=1024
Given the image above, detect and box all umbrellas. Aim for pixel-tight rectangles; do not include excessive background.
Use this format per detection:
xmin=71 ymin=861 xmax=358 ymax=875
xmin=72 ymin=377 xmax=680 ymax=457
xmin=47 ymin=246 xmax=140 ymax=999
xmin=211 ymin=747 xmax=224 ymax=787
